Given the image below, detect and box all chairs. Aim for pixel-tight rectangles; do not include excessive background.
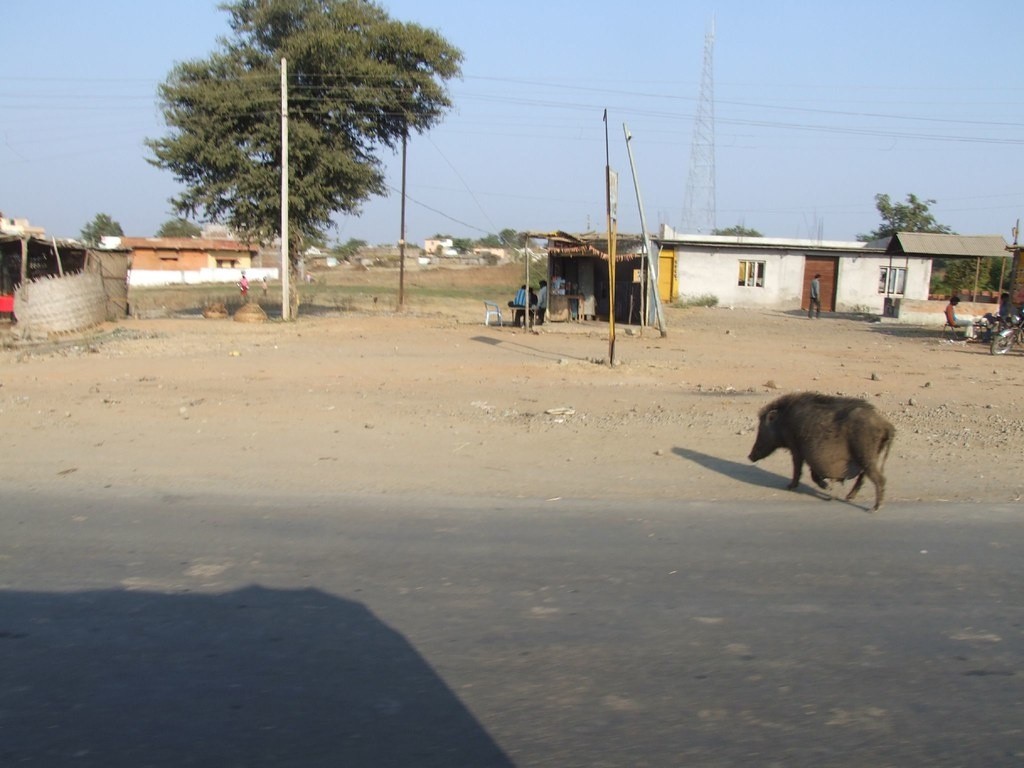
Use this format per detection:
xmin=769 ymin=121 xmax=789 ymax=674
xmin=483 ymin=301 xmax=503 ymax=327
xmin=941 ymin=311 xmax=960 ymax=341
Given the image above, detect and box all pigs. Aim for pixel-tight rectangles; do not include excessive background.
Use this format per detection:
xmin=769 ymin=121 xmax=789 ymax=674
xmin=748 ymin=391 xmax=898 ymax=513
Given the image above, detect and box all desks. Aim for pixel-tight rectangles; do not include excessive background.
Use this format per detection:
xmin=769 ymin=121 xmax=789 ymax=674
xmin=552 ymin=296 xmax=584 ymax=323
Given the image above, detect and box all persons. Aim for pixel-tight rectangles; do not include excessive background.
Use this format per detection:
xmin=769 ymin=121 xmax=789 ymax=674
xmin=999 ymin=293 xmax=1016 ymax=317
xmin=946 ymin=297 xmax=977 ymax=341
xmin=807 ymin=273 xmax=822 ymax=319
xmin=237 ymin=270 xmax=250 ymax=303
xmin=508 ymin=280 xmax=547 ymax=328
xmin=262 ymin=278 xmax=268 ymax=297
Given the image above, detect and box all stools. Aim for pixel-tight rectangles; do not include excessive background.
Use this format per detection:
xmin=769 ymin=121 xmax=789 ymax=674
xmin=509 ymin=307 xmax=538 ymax=328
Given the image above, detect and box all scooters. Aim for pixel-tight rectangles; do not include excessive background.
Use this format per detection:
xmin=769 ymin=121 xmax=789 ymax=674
xmin=989 ymin=308 xmax=1024 ymax=356
